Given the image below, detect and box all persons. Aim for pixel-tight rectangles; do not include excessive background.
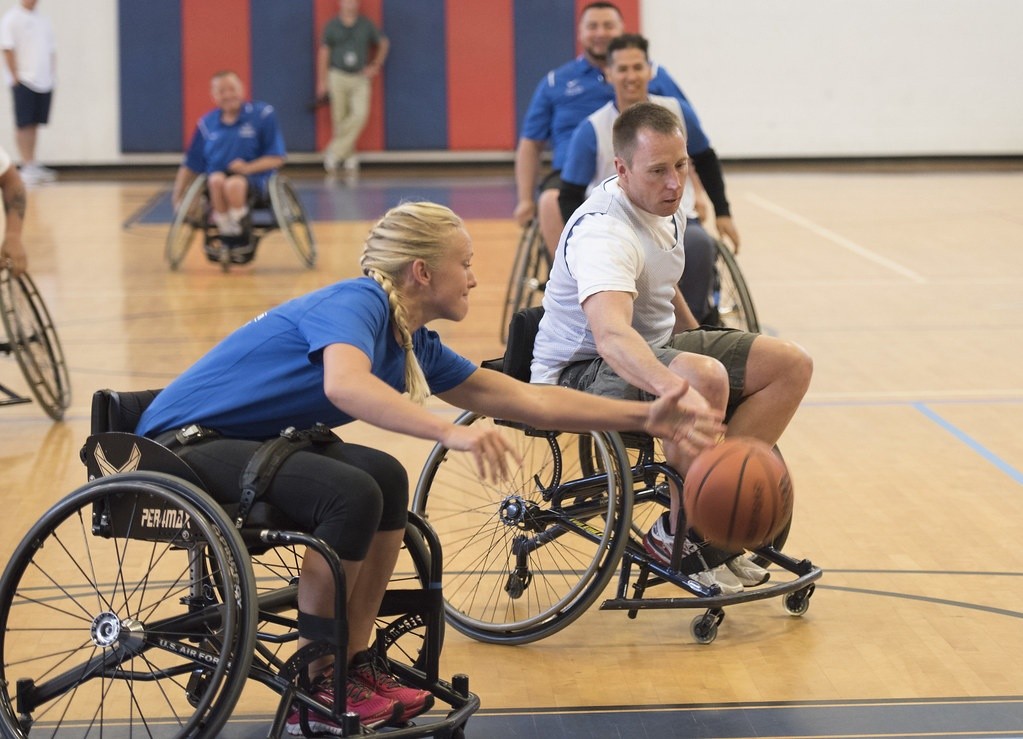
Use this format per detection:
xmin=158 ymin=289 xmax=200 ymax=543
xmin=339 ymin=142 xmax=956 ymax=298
xmin=172 ymin=70 xmax=287 ymax=245
xmin=315 ymin=0 xmax=391 ymax=176
xmin=514 ymin=3 xmax=740 ymax=256
xmin=135 ymin=202 xmax=728 ymax=736
xmin=558 ymin=33 xmax=714 ymax=331
xmin=528 ymin=105 xmax=815 ymax=592
xmin=0 ymin=0 xmax=55 ymax=186
xmin=0 ymin=146 xmax=27 ymax=277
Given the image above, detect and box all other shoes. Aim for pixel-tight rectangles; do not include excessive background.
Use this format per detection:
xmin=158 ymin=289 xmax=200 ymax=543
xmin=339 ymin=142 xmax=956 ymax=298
xmin=18 ymin=164 xmax=57 ymax=184
xmin=208 ymin=230 xmax=254 ymax=263
xmin=322 ymin=147 xmax=339 ymax=173
xmin=342 ymin=152 xmax=362 ymax=177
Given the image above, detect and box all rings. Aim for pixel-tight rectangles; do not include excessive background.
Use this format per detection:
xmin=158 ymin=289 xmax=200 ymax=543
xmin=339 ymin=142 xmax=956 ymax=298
xmin=687 ymin=431 xmax=694 ymax=439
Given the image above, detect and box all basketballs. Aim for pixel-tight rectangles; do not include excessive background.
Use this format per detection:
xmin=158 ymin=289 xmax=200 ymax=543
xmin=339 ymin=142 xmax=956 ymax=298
xmin=680 ymin=435 xmax=795 ymax=554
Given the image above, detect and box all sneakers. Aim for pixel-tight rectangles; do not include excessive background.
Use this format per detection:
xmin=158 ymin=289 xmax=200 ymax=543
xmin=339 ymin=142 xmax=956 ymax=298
xmin=285 ymin=679 xmax=404 ymax=735
xmin=726 ymin=555 xmax=770 ymax=587
xmin=350 ymin=662 xmax=434 ymax=721
xmin=643 ymin=511 xmax=744 ymax=596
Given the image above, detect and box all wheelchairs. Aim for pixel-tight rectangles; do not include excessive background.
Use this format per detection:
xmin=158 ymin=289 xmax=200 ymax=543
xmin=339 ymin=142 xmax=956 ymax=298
xmin=161 ymin=173 xmax=319 ymax=274
xmin=0 ymin=257 xmax=75 ymax=425
xmin=496 ymin=215 xmax=770 ymax=353
xmin=0 ymin=390 xmax=487 ymax=739
xmin=410 ymin=306 xmax=826 ymax=649
